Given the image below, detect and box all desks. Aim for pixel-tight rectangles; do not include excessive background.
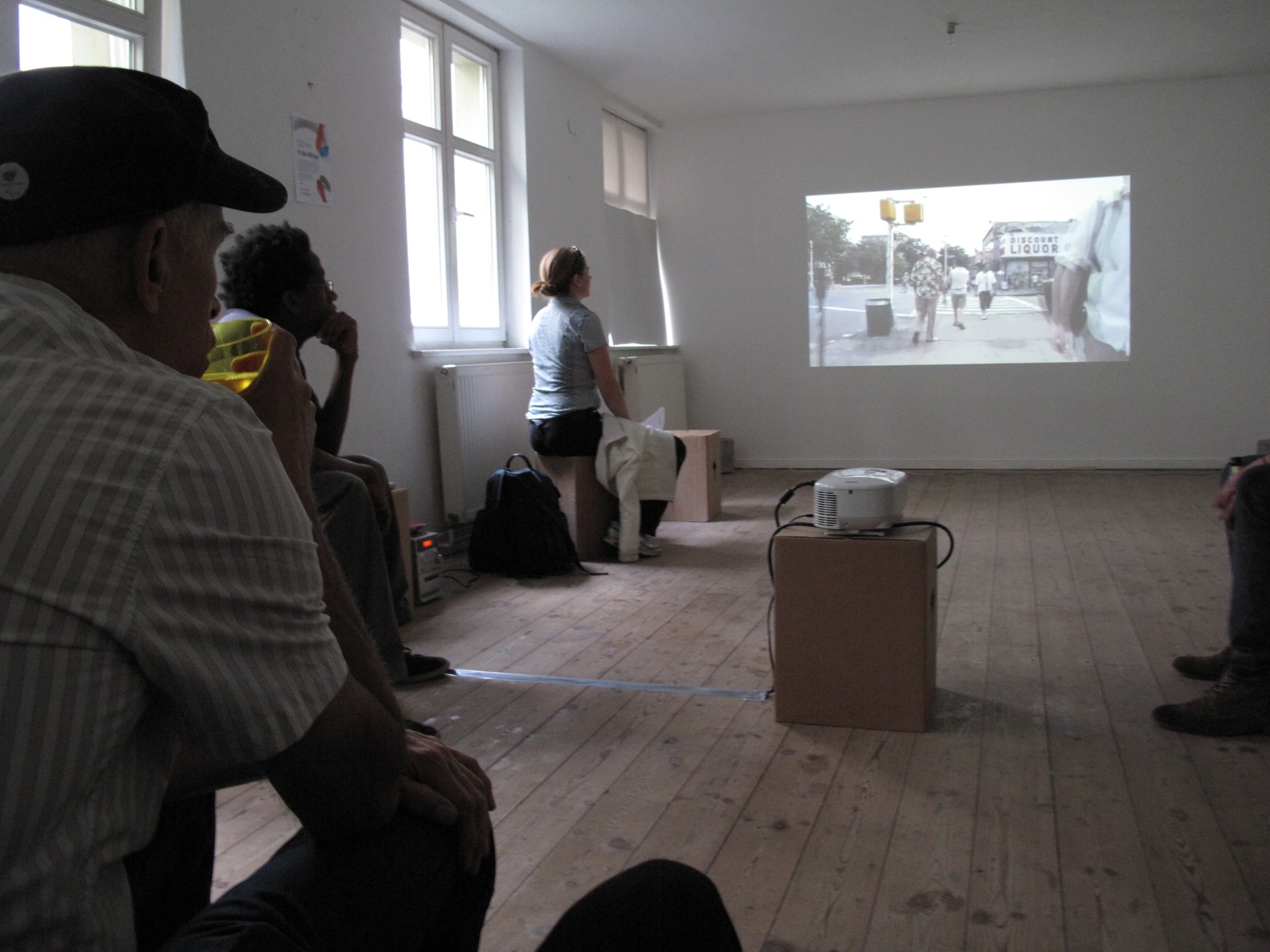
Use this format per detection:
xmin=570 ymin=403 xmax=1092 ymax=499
xmin=663 ymin=430 xmax=721 ymax=523
xmin=773 ymin=517 xmax=942 ymax=731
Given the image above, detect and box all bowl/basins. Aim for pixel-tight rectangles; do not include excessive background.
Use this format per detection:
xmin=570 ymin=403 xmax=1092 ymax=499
xmin=201 ymin=319 xmax=273 ymax=394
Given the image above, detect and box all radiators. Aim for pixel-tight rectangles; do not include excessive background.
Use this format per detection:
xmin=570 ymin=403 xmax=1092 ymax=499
xmin=615 ymin=354 xmax=686 ymax=429
xmin=437 ymin=360 xmax=536 ymax=520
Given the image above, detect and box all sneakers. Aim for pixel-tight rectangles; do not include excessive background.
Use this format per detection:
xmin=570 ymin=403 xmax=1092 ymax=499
xmin=605 ymin=522 xmax=663 ymax=557
xmin=1174 ymin=643 xmax=1232 ymax=681
xmin=1152 ymin=667 xmax=1270 ymax=737
xmin=399 ymin=647 xmax=449 ymax=684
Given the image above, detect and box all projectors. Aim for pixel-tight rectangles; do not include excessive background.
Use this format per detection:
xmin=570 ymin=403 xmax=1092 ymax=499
xmin=812 ymin=466 xmax=909 ymax=533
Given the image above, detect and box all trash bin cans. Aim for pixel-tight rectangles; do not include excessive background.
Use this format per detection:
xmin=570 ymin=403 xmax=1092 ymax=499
xmin=864 ymin=297 xmax=893 ymax=337
xmin=1257 ymin=440 xmax=1270 ymax=454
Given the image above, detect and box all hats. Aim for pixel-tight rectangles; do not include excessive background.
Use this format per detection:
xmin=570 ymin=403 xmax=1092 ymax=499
xmin=0 ymin=67 xmax=288 ymax=244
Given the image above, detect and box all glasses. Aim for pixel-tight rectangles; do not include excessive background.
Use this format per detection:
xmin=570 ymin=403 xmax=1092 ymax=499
xmin=310 ymin=280 xmax=334 ymax=293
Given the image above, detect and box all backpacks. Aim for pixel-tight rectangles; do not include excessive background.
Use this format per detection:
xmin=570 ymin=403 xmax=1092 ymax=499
xmin=468 ymin=454 xmax=609 ymax=578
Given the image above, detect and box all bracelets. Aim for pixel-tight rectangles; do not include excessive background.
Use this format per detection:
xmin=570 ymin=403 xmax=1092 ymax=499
xmin=1263 ymin=455 xmax=1266 ymax=464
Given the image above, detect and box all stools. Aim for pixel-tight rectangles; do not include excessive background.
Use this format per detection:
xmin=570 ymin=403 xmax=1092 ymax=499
xmin=532 ymin=452 xmax=611 ymax=559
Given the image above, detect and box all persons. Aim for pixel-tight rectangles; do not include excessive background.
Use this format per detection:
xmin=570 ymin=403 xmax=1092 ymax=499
xmin=0 ymin=63 xmax=740 ymax=952
xmin=1032 ymin=273 xmax=1039 ymax=288
xmin=1051 ymin=175 xmax=1130 ymax=360
xmin=1150 ymin=452 xmax=1270 ymax=738
xmin=527 ymin=246 xmax=686 ymax=557
xmin=911 ymin=248 xmax=946 ymax=343
xmin=947 ymin=258 xmax=971 ymax=329
xmin=900 ymin=272 xmax=909 ymax=294
xmin=975 ymin=263 xmax=996 ymax=319
xmin=218 ymin=221 xmax=449 ymax=687
